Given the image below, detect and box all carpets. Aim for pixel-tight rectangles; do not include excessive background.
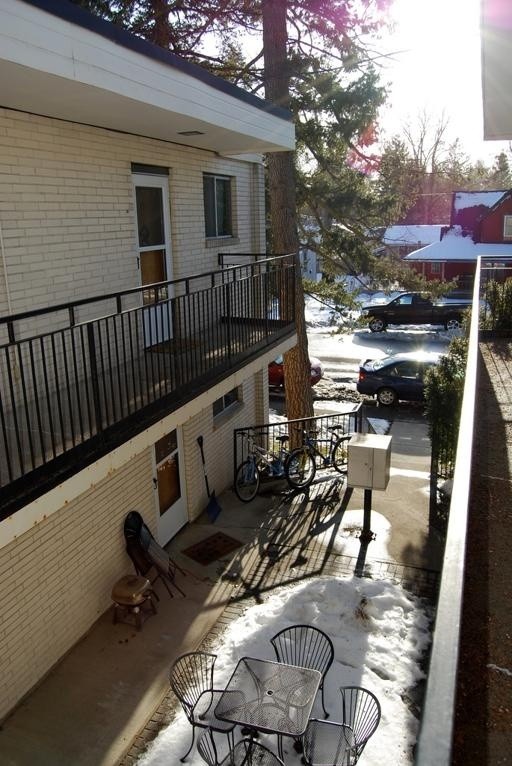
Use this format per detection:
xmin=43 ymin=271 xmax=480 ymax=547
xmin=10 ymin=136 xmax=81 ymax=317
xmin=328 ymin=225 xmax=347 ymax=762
xmin=180 ymin=531 xmax=247 ymax=567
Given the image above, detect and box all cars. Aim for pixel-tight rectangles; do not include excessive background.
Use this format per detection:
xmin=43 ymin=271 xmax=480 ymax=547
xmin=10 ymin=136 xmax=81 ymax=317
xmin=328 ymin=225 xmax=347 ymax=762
xmin=269 ymin=358 xmax=324 ymax=386
xmin=357 ymin=353 xmax=439 ymax=406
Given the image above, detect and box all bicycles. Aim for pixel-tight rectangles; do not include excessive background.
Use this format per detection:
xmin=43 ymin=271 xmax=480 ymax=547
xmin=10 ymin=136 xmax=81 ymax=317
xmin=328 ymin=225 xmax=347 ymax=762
xmin=234 ymin=432 xmax=316 ymax=503
xmin=285 ymin=425 xmax=352 ymax=489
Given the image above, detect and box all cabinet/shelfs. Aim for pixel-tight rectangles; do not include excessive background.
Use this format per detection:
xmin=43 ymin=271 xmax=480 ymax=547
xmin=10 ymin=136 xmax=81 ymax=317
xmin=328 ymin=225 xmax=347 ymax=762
xmin=347 ymin=433 xmax=392 ymax=491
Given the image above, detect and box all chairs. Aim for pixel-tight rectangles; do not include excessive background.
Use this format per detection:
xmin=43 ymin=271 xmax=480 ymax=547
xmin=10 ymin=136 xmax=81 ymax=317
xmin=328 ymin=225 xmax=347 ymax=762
xmin=270 ymin=625 xmax=335 ymax=720
xmin=303 ymin=686 xmax=381 ymax=765
xmin=144 ymin=536 xmax=187 ymax=600
xmin=219 ymin=738 xmax=285 ymax=765
xmin=169 ymin=651 xmax=246 ymax=763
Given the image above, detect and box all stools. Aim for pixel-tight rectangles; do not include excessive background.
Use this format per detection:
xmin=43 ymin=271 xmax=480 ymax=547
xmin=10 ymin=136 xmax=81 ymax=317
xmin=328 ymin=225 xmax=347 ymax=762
xmin=111 ymin=574 xmax=160 ymax=632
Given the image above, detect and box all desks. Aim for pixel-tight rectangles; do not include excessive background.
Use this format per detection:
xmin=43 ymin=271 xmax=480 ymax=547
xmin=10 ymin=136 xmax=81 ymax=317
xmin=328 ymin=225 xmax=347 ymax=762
xmin=215 ymin=657 xmax=322 ymax=765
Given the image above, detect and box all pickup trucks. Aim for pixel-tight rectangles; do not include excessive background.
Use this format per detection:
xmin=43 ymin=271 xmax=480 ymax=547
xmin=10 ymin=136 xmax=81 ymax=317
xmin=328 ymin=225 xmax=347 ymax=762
xmin=362 ymin=293 xmax=472 ymax=332
